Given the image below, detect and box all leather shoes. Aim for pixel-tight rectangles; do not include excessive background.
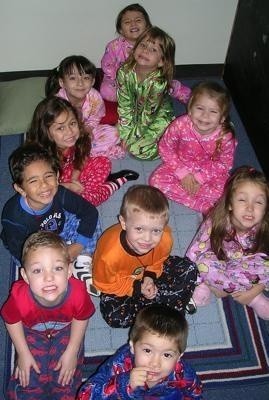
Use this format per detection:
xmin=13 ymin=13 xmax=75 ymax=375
xmin=105 ymin=169 xmax=139 ymax=182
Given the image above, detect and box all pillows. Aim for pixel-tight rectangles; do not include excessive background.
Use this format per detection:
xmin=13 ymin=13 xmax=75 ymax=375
xmin=1 ymin=76 xmax=58 ymax=135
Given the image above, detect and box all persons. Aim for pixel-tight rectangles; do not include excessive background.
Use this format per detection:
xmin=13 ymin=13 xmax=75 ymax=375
xmin=184 ymin=166 xmax=269 ymax=321
xmin=99 ymin=3 xmax=191 ymax=105
xmin=149 ymin=81 xmax=238 ymax=216
xmin=92 ymin=184 xmax=200 ymax=328
xmin=0 ymin=230 xmax=95 ymax=400
xmin=0 ymin=142 xmax=102 ymax=298
xmin=25 ymin=96 xmax=140 ymax=207
xmin=45 ymin=54 xmax=126 ymax=160
xmin=114 ymin=26 xmax=177 ymax=161
xmin=77 ymin=304 xmax=204 ymax=400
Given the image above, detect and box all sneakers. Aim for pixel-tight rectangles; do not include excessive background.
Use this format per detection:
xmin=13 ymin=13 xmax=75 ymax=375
xmin=71 ymin=262 xmax=104 ymax=297
xmin=186 ymin=298 xmax=197 ymax=314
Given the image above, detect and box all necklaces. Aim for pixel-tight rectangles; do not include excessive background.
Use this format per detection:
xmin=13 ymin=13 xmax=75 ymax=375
xmin=36 ymin=302 xmax=62 ymax=339
xmin=130 ymin=247 xmax=155 ymax=268
